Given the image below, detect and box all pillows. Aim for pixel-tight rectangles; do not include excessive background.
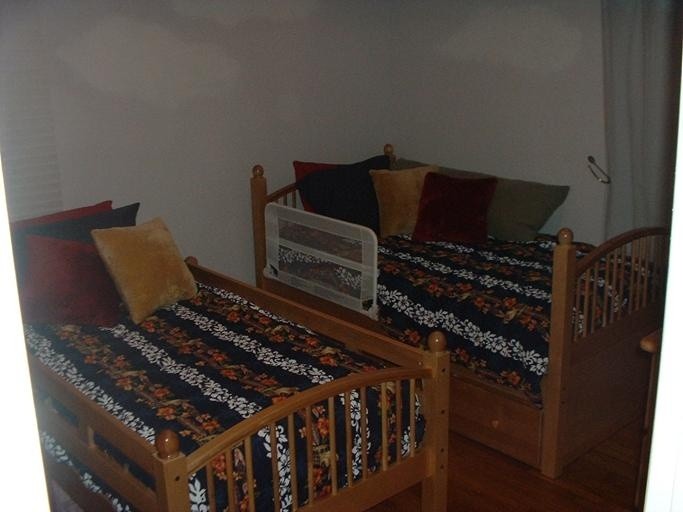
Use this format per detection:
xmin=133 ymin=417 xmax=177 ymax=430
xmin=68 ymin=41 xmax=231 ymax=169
xmin=9 ymin=198 xmax=197 ymax=329
xmin=289 ymin=158 xmax=337 ymax=215
xmin=295 ymin=154 xmax=389 ymax=237
xmin=366 ymin=162 xmax=440 ymax=239
xmin=395 ymin=155 xmax=566 ymax=243
xmin=411 ymin=172 xmax=497 ymax=245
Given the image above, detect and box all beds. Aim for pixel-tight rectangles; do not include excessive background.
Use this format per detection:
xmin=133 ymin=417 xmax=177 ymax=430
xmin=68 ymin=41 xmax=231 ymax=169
xmin=23 ymin=257 xmax=451 ymax=511
xmin=250 ymin=144 xmax=669 ymax=482
